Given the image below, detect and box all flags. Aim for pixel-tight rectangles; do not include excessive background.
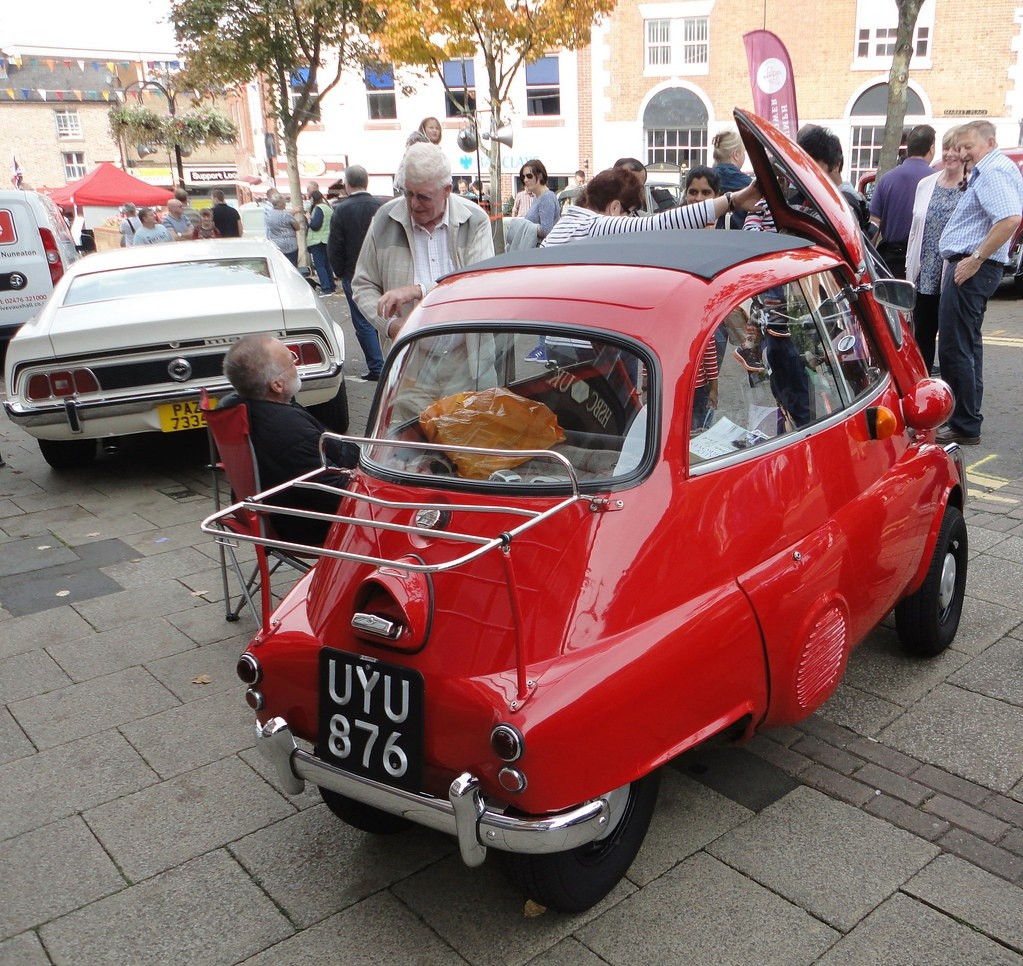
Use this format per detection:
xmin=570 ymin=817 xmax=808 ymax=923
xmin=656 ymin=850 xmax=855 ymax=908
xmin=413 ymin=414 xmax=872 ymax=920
xmin=10 ymin=156 xmax=23 ymax=190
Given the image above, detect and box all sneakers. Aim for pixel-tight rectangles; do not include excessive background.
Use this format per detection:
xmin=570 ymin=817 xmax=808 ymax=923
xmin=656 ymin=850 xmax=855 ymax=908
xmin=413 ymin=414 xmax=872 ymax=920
xmin=524 ymin=347 xmax=543 ymax=361
xmin=537 ymin=353 xmax=547 ymax=363
xmin=768 ymin=322 xmax=792 ymax=338
xmin=733 ymin=345 xmax=766 ymax=372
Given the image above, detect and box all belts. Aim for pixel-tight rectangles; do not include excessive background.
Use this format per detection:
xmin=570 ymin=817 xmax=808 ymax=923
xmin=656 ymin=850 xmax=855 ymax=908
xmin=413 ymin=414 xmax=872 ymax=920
xmin=948 ymin=254 xmax=1004 ymax=268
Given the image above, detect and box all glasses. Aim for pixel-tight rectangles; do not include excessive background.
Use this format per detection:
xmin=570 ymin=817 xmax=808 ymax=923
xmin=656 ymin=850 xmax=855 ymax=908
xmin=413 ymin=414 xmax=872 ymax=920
xmin=621 ymin=203 xmax=634 ymax=217
xmin=521 ymin=174 xmax=535 ymax=180
xmin=275 ymin=351 xmax=299 ymax=379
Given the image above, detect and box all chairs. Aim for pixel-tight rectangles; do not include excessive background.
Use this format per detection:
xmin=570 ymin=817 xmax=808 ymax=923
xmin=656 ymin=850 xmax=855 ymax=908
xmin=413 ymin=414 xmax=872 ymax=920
xmin=198 ymin=385 xmax=313 ymax=629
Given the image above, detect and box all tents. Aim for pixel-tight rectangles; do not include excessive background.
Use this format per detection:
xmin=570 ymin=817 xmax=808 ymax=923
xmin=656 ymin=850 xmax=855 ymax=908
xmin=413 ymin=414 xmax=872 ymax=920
xmin=48 ymin=161 xmax=175 ymax=216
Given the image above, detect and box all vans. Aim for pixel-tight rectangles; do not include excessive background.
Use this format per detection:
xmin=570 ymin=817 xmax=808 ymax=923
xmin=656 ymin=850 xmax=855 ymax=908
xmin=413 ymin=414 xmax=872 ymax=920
xmin=0 ymin=189 xmax=96 ymax=363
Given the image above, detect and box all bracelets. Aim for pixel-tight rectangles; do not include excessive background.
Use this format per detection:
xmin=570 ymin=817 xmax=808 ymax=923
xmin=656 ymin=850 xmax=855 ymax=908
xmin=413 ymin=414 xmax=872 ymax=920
xmin=725 ymin=192 xmax=734 ymax=212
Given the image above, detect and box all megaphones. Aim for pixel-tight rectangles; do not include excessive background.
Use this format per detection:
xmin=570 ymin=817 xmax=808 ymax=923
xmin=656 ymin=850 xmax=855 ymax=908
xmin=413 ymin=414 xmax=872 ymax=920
xmin=137 ymin=143 xmax=157 ymax=158
xmin=180 ymin=145 xmax=192 ymax=157
xmin=457 ymin=127 xmax=478 ymax=152
xmin=482 ymin=123 xmax=513 ymax=148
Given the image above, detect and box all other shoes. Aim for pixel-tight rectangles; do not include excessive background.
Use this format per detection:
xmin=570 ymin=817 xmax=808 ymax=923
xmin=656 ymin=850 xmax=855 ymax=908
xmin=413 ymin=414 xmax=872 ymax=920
xmin=932 ymin=366 xmax=940 ymax=376
xmin=361 ymin=372 xmax=378 ymax=381
xmin=318 ymin=290 xmax=337 ymax=298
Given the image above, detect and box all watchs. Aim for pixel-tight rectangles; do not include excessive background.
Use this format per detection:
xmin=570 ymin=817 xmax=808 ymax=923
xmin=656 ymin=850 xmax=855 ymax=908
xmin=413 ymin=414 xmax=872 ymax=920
xmin=973 ymin=250 xmax=985 ymax=262
xmin=177 ymin=232 xmax=182 ymax=236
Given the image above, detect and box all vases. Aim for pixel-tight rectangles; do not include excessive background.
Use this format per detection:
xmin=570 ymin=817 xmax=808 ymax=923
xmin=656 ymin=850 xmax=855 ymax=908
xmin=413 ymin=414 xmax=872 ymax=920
xmin=180 ymin=123 xmax=222 ymax=139
xmin=121 ymin=118 xmax=157 ymax=130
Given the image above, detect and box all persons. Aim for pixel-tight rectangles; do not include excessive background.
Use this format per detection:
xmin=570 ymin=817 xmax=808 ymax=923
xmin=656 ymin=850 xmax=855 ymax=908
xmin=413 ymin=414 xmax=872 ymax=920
xmin=214 ymin=334 xmax=366 ymax=551
xmin=267 ymin=117 xmax=1023 ymax=445
xmin=58 ymin=189 xmax=244 ymax=247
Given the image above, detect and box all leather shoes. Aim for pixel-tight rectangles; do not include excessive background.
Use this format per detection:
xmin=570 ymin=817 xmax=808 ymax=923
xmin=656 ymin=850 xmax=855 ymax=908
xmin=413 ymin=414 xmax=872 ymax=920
xmin=935 ymin=424 xmax=981 ymax=445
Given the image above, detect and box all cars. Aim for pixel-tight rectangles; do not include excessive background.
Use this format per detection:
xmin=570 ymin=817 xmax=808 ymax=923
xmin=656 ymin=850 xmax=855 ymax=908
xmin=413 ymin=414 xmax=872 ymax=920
xmin=3 ymin=233 xmax=350 ymax=468
xmin=857 ymin=145 xmax=1023 ymax=293
xmin=199 ymin=107 xmax=970 ymax=912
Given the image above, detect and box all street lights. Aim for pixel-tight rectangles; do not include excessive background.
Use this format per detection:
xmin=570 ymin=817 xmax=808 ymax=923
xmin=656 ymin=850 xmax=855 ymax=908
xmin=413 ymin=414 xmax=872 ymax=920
xmin=116 ymin=81 xmax=216 ymax=203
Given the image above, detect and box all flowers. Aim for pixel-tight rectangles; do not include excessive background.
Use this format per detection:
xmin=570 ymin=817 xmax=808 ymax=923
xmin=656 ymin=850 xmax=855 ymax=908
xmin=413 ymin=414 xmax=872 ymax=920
xmin=107 ymin=102 xmax=239 ymax=156
